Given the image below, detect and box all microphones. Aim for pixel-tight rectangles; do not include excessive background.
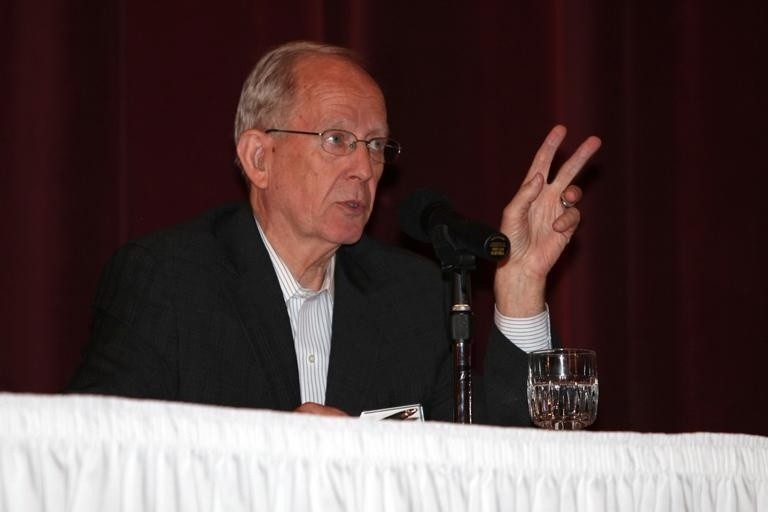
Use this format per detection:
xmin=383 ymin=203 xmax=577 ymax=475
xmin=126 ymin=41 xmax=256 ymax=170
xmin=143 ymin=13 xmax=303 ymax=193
xmin=399 ymin=189 xmax=510 ymax=261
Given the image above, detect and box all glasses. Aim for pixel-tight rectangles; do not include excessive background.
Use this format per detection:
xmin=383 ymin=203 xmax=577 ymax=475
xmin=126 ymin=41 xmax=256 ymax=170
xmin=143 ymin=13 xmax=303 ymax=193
xmin=265 ymin=129 xmax=401 ymax=164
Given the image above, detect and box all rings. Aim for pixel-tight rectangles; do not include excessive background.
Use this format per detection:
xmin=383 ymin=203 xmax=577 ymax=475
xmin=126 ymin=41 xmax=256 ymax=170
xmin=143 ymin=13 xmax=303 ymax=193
xmin=560 ymin=194 xmax=575 ymax=208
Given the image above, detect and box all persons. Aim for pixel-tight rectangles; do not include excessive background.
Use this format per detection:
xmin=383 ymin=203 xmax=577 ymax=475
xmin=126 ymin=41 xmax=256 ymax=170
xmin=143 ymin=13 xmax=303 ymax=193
xmin=61 ymin=41 xmax=603 ymax=427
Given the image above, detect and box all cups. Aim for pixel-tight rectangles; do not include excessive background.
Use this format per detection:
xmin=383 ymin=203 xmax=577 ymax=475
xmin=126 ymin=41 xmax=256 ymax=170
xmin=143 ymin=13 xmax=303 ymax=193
xmin=527 ymin=348 xmax=599 ymax=430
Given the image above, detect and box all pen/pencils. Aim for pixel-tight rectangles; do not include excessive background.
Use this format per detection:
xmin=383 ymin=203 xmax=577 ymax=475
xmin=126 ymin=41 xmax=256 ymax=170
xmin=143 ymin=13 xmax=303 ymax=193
xmin=382 ymin=408 xmax=417 ymax=420
